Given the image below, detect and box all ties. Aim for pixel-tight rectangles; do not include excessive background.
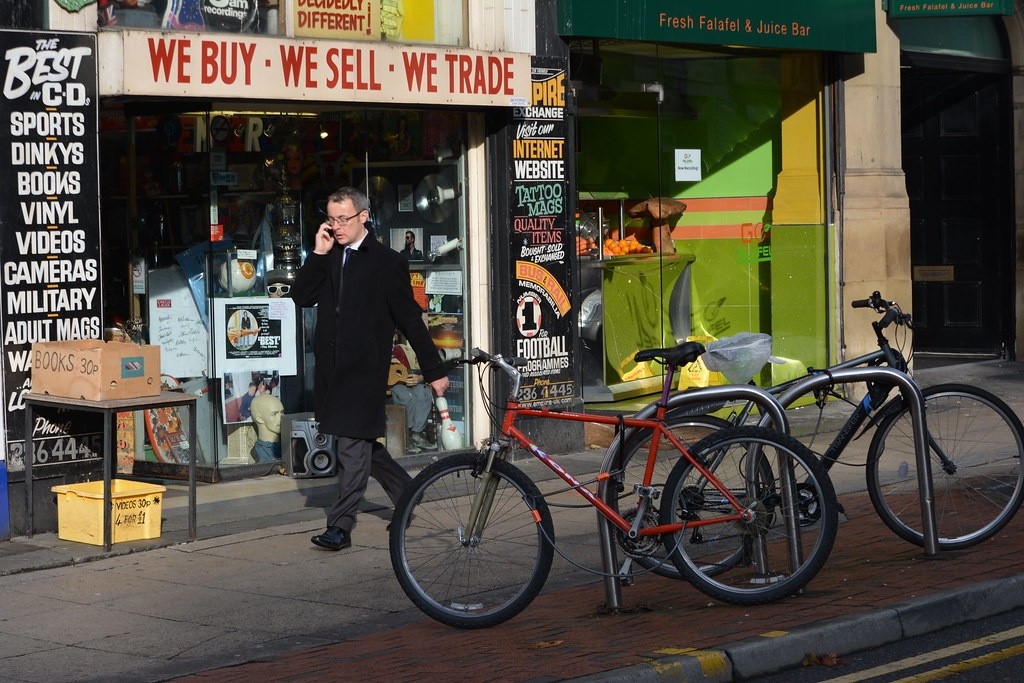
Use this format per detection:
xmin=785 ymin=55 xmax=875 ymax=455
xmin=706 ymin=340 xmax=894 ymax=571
xmin=342 ymin=248 xmax=351 ymax=280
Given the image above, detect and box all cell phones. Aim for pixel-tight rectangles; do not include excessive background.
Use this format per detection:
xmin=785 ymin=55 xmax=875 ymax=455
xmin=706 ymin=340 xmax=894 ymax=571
xmin=327 ymin=224 xmax=333 ymax=238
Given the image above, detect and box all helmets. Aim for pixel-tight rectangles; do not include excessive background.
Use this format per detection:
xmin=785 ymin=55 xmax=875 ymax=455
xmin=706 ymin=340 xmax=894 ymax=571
xmin=263 ymin=269 xmax=293 ymax=295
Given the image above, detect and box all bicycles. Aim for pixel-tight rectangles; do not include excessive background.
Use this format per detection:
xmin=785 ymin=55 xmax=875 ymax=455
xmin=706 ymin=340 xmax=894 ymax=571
xmin=389 ymin=344 xmax=838 ymax=632
xmin=605 ymin=290 xmax=1023 ymax=580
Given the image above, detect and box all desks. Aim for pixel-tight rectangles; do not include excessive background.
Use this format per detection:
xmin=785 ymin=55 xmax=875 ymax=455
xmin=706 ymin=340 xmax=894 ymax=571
xmin=23 ymin=390 xmax=199 ymax=553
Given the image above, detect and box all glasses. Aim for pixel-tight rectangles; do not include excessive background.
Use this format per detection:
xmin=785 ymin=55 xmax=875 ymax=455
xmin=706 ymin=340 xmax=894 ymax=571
xmin=266 ymin=285 xmax=291 ymax=295
xmin=325 ymin=209 xmax=365 ymax=226
xmin=406 ymin=236 xmax=411 ymax=240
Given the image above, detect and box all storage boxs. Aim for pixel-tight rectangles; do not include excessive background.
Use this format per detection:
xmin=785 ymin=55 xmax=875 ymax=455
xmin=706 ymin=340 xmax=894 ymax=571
xmin=31 ymin=339 xmax=162 ymax=402
xmin=51 ymin=479 xmax=167 ymax=547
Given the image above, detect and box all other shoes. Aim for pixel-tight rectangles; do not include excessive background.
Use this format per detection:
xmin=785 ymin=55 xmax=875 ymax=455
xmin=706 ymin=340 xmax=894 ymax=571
xmin=406 ymin=430 xmax=438 ymax=453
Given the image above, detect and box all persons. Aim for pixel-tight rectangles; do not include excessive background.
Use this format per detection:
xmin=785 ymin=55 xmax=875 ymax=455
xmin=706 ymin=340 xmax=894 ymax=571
xmin=241 ymin=311 xmax=251 ymax=348
xmin=239 ymin=378 xmax=278 ymax=419
xmin=399 ymin=231 xmax=423 ymax=260
xmin=251 ymin=394 xmax=286 ymax=463
xmin=390 ymin=336 xmax=438 ymax=453
xmin=289 ymin=186 xmax=449 ymax=551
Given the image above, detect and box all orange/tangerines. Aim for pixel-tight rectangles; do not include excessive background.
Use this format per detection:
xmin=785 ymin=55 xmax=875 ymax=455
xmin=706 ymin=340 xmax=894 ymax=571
xmin=603 ymin=239 xmax=654 ymax=256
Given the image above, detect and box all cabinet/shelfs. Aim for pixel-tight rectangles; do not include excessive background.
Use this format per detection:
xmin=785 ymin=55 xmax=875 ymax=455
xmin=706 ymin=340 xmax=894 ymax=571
xmin=110 ymin=156 xmax=277 ymax=260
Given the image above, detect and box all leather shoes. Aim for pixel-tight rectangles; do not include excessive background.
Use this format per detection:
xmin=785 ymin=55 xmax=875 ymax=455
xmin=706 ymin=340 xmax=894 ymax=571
xmin=386 ymin=491 xmax=423 ymax=531
xmin=311 ymin=526 xmax=352 ymax=550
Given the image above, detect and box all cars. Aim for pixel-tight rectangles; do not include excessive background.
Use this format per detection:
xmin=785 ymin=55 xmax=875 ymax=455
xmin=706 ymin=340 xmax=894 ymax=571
xmin=578 ymin=285 xmax=604 ymax=357
